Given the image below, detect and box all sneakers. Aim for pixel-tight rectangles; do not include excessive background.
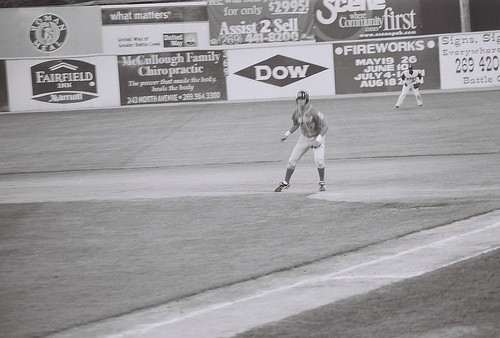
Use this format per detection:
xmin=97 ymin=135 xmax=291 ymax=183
xmin=274 ymin=182 xmax=290 ymax=192
xmin=319 ymin=182 xmax=326 ymax=191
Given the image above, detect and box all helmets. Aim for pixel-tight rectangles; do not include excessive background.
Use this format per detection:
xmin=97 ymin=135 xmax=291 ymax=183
xmin=296 ymin=91 xmax=309 ymax=104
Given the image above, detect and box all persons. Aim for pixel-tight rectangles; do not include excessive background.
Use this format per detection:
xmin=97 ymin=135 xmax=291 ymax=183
xmin=395 ymin=66 xmax=423 ymax=107
xmin=275 ymin=91 xmax=328 ymax=194
